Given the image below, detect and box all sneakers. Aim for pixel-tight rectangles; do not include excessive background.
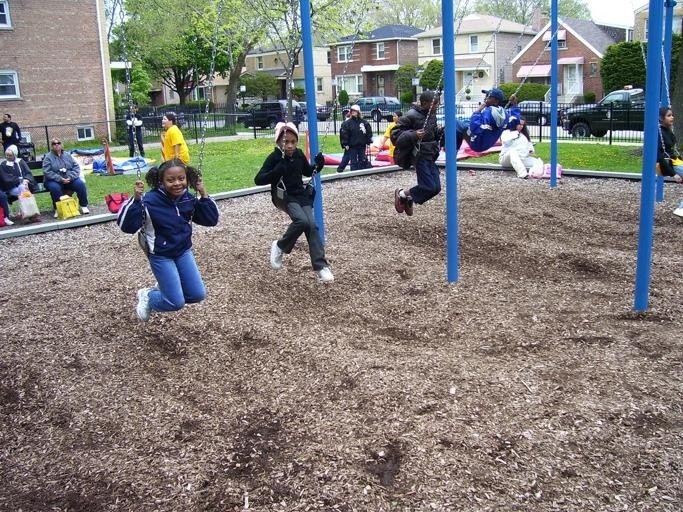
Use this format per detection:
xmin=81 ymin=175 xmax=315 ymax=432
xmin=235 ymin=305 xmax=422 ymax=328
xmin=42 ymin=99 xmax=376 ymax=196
xmin=3 ymin=217 xmax=14 ymax=226
xmin=673 ymin=207 xmax=683 ymax=217
xmin=80 ymin=206 xmax=89 ymax=214
xmin=136 ymin=289 xmax=154 ymax=322
xmin=270 ymin=239 xmax=283 ymax=271
xmin=317 ymin=264 xmax=334 ymax=285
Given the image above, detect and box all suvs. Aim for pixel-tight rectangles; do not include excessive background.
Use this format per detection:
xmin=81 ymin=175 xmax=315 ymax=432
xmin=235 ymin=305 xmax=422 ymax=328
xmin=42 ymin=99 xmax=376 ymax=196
xmin=343 ymin=96 xmax=401 ymax=122
xmin=236 ymin=99 xmax=304 ymax=130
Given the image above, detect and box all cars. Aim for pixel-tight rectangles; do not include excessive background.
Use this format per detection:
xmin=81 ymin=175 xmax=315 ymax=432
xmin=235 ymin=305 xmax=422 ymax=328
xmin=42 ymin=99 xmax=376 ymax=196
xmin=435 ymin=102 xmax=485 ymax=140
xmin=298 ymin=101 xmax=330 ymax=122
xmin=510 ymin=100 xmax=566 ymax=127
xmin=139 ymin=108 xmax=186 ymax=130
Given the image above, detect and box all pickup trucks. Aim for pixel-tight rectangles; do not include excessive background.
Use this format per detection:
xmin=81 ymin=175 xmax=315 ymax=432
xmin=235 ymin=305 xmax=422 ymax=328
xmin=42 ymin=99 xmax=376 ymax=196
xmin=562 ymin=86 xmax=671 ymax=139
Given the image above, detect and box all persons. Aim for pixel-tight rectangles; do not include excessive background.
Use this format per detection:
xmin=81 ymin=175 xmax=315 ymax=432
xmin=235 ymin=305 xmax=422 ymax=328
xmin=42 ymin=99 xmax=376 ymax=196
xmin=117 ymin=159 xmax=219 ymax=322
xmin=0 ymin=114 xmax=42 ymax=226
xmin=42 ymin=138 xmax=89 ymax=219
xmin=125 ymin=104 xmax=145 ymax=158
xmin=657 ymin=106 xmax=683 ymax=217
xmin=161 ymin=112 xmax=191 ymax=165
xmin=254 ymin=122 xmax=335 ymax=285
xmin=336 ymin=87 xmax=536 ymax=216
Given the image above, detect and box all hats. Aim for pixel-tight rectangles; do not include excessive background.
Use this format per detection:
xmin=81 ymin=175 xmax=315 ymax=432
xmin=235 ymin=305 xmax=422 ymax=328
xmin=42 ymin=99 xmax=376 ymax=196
xmin=350 ymin=104 xmax=361 ymax=113
xmin=7 ymin=144 xmax=19 ymax=155
xmin=482 ymin=87 xmax=503 ymax=102
xmin=275 ymin=122 xmax=299 ymax=146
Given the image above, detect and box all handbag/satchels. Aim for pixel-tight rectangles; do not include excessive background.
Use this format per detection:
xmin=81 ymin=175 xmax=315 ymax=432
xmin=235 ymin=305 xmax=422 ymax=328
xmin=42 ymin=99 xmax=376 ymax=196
xmin=55 ymin=197 xmax=80 ymax=222
xmin=17 ymin=191 xmax=41 ymax=221
xmin=543 ymin=163 xmax=563 ymax=178
xmin=103 ymin=193 xmax=128 ymax=212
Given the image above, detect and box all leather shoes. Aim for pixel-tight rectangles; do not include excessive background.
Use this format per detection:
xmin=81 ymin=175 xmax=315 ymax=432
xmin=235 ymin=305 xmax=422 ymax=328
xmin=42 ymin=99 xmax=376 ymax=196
xmin=402 ymin=190 xmax=414 ymax=216
xmin=395 ymin=188 xmax=406 ymax=213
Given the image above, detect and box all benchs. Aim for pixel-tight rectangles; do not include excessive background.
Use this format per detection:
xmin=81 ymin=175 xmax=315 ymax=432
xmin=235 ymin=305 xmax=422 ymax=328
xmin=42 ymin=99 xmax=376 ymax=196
xmin=26 ymin=160 xmax=73 ymax=197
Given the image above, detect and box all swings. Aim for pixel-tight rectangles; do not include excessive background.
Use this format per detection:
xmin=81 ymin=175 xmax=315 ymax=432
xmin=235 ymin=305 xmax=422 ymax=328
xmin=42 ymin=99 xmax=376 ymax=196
xmin=116 ymin=0 xmax=224 ymax=257
xmin=632 ymin=0 xmax=681 ymax=177
xmin=276 ymin=1 xmax=368 ymax=212
xmin=411 ymin=0 xmax=577 ymax=169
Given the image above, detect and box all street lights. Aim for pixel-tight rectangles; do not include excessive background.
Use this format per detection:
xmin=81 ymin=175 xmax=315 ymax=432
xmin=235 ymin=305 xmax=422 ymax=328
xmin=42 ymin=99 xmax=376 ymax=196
xmin=239 ymin=84 xmax=249 ymax=105
